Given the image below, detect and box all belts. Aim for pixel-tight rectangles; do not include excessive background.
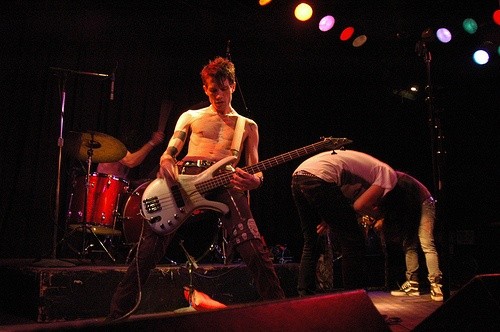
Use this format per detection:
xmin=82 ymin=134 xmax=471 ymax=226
xmin=183 ymin=159 xmax=214 ymax=169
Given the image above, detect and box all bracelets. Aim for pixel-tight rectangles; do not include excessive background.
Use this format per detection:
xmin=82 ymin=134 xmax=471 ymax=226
xmin=255 ymin=174 xmax=264 ymax=189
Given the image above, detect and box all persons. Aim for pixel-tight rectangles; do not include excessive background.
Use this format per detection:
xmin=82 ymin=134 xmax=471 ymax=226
xmin=96 ymin=127 xmax=165 ymax=180
xmin=107 ymin=57 xmax=285 ymax=320
xmin=292 ymin=149 xmax=445 ymax=301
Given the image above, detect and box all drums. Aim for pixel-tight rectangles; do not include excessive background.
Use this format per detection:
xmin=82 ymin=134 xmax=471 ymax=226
xmin=122 ymin=181 xmax=221 ymax=266
xmin=65 ymin=171 xmax=129 ymax=235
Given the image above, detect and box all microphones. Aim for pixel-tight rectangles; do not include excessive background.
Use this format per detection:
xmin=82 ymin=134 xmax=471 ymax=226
xmin=108 ymin=69 xmax=116 ymax=101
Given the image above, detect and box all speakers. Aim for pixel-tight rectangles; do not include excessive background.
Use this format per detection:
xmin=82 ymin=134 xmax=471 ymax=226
xmin=63 ymin=287 xmax=394 ymax=331
xmin=411 ymin=272 xmax=500 ymax=332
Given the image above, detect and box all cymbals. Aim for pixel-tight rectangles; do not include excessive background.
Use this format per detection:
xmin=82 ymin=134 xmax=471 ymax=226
xmin=63 ymin=130 xmax=128 ymax=162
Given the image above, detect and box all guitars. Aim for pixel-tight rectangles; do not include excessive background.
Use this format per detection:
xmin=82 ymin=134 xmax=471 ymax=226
xmin=142 ymin=136 xmax=353 ymax=237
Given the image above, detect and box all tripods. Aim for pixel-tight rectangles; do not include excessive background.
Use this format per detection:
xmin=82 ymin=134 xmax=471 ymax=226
xmin=54 ymin=140 xmax=116 ymax=266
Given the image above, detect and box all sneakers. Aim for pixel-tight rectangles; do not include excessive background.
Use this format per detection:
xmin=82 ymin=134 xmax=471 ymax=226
xmin=430 ymin=283 xmax=445 ymax=301
xmin=389 ymin=281 xmax=421 ymax=297
